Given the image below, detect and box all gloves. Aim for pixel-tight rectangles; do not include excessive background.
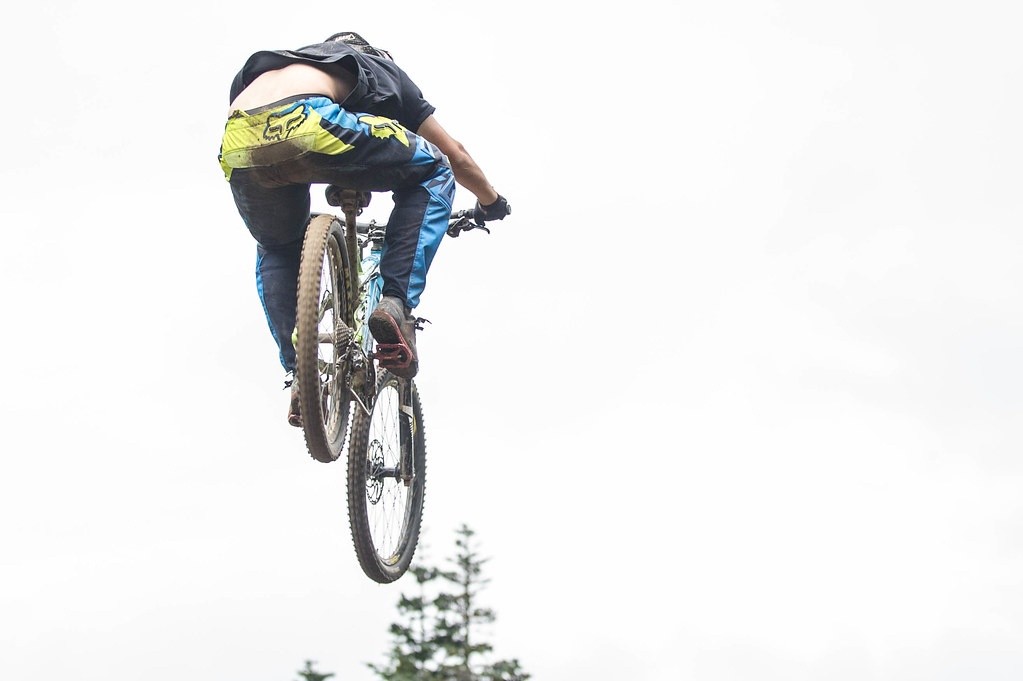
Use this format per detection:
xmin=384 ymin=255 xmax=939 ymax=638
xmin=473 ymin=193 xmax=507 ymax=225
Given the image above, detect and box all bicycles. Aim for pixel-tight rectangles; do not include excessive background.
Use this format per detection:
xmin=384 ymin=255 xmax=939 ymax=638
xmin=296 ymin=179 xmax=512 ymax=582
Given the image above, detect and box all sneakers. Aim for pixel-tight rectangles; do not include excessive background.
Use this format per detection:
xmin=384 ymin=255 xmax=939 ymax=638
xmin=286 ymin=386 xmax=303 ymax=428
xmin=367 ymin=296 xmax=419 ymax=379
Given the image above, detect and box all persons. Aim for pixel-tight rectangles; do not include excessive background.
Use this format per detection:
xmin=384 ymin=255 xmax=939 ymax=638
xmin=219 ymin=32 xmax=508 ymax=427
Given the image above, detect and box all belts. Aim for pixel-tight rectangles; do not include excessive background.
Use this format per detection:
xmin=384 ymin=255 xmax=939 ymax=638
xmin=229 ymin=93 xmax=334 ymax=120
